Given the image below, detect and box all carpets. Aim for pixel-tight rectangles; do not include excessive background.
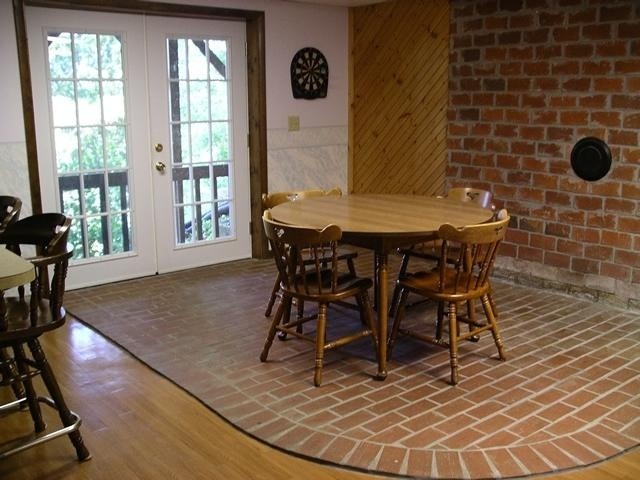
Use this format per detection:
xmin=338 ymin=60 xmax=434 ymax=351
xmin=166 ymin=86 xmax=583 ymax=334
xmin=63 ymin=246 xmax=640 ymax=480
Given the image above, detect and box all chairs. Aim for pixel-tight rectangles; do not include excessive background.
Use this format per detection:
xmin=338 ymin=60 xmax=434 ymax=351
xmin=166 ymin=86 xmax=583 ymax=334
xmin=0 ymin=213 xmax=91 ymax=461
xmin=397 ymin=187 xmax=498 ymax=334
xmin=382 ymin=209 xmax=506 ymax=384
xmin=259 ymin=211 xmax=379 ymax=384
xmin=0 ymin=194 xmax=31 ymax=412
xmin=262 ymin=188 xmax=356 ymax=316
